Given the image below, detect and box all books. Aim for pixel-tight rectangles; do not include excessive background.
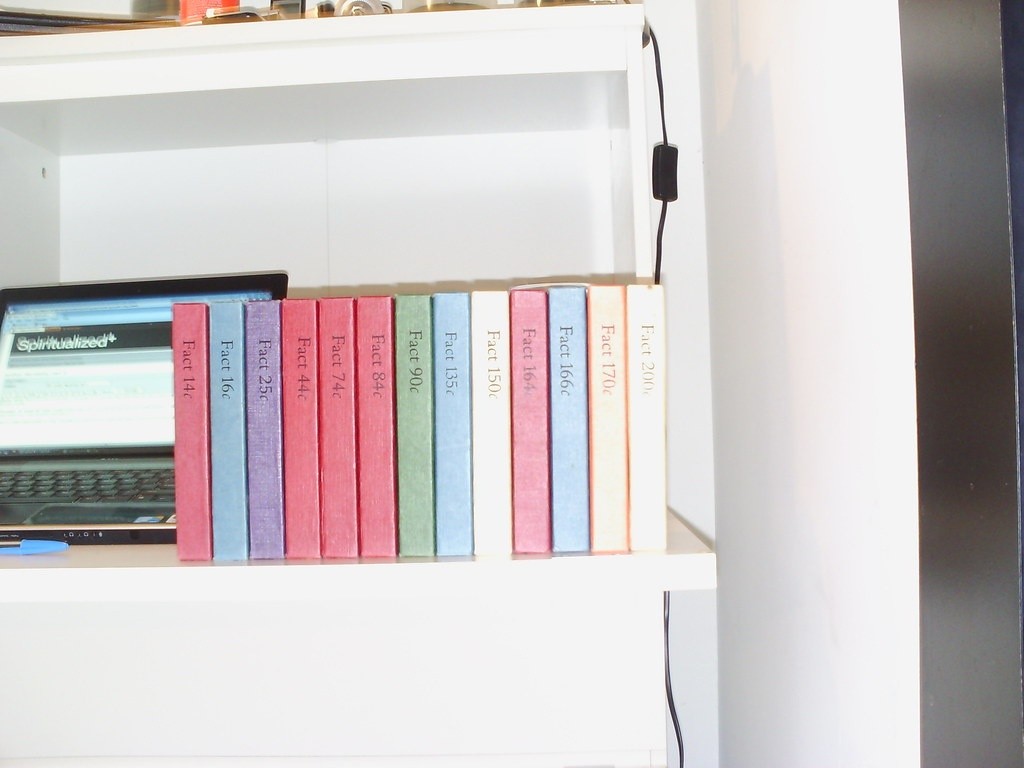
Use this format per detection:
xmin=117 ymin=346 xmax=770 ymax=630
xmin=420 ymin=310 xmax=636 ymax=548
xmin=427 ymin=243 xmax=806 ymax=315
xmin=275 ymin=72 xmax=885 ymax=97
xmin=171 ymin=285 xmax=671 ymax=563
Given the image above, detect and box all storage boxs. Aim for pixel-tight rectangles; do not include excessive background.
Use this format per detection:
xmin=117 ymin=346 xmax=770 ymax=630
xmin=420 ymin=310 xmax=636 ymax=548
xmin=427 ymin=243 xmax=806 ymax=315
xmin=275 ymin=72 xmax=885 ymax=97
xmin=0 ymin=0 xmax=181 ymax=22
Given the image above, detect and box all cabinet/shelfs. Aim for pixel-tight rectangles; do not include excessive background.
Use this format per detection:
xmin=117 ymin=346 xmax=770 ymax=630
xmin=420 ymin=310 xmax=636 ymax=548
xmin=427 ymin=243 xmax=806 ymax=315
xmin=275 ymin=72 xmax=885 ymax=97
xmin=0 ymin=0 xmax=717 ymax=606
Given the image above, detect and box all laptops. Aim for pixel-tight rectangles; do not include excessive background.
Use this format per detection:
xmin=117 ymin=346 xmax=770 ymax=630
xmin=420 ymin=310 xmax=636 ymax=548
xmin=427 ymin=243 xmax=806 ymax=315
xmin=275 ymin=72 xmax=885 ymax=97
xmin=0 ymin=270 xmax=288 ymax=549
